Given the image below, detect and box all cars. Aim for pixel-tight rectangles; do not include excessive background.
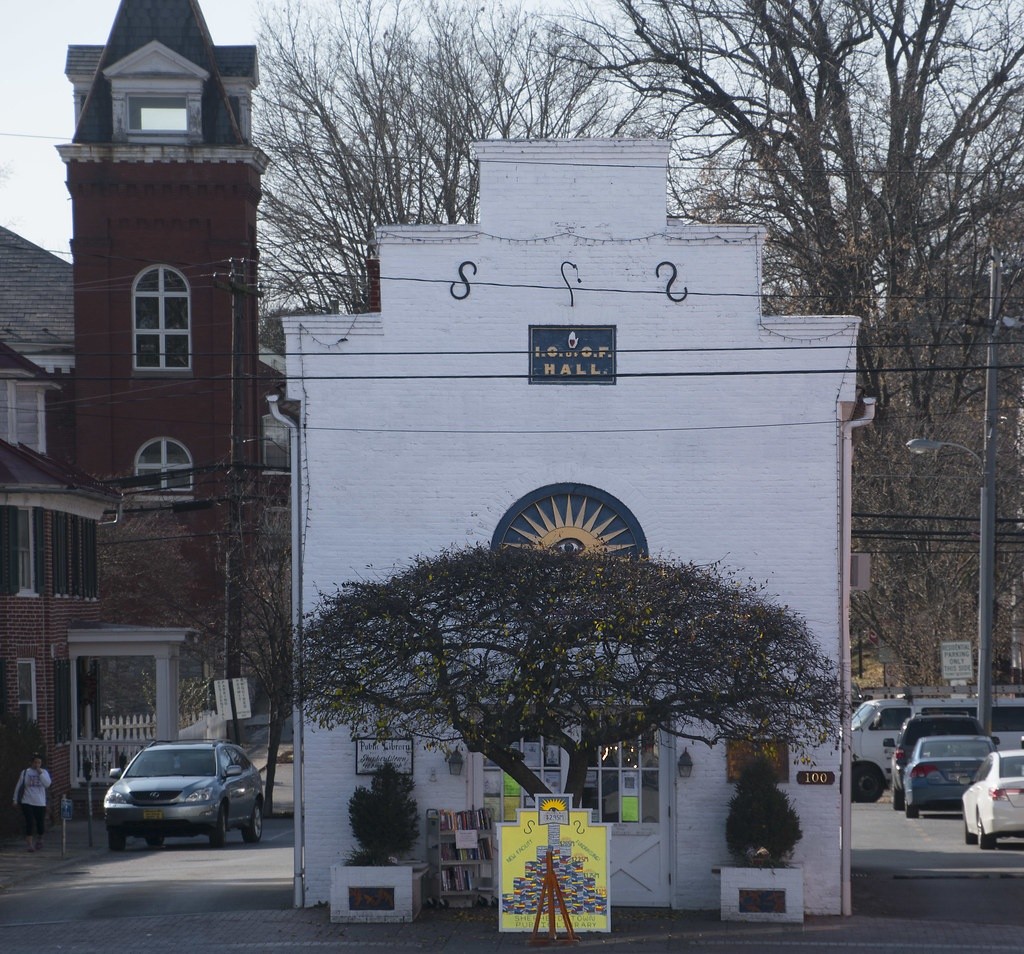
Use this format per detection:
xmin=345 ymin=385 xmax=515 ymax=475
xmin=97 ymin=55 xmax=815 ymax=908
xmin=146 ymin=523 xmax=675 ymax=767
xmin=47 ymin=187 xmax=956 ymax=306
xmin=895 ymin=735 xmax=998 ymax=819
xmin=963 ymin=749 xmax=1024 ymax=850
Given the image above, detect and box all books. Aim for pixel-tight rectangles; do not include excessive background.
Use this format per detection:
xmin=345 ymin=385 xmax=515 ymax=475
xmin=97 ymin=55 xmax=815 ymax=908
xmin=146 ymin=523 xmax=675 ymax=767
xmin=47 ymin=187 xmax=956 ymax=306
xmin=432 ymin=806 xmax=494 ymax=893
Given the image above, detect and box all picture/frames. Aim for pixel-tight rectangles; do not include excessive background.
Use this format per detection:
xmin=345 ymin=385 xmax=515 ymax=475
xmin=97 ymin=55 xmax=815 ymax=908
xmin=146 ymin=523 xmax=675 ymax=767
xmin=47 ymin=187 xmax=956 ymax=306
xmin=354 ymin=735 xmax=413 ymax=776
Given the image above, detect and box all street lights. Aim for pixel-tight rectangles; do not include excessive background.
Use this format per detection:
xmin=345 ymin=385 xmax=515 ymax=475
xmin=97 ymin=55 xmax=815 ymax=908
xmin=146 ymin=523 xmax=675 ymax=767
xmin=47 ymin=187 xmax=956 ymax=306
xmin=907 ymin=438 xmax=994 ymax=736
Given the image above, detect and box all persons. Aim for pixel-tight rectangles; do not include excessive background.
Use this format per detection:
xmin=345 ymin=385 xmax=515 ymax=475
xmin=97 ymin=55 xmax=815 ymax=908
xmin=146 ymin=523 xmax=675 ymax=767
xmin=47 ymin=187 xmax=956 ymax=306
xmin=12 ymin=755 xmax=52 ymax=852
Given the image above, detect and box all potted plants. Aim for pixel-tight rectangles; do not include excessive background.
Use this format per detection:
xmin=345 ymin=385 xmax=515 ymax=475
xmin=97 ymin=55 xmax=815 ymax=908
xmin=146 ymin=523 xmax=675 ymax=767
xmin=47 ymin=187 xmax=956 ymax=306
xmin=719 ymin=756 xmax=807 ymax=926
xmin=331 ymin=764 xmax=423 ymax=924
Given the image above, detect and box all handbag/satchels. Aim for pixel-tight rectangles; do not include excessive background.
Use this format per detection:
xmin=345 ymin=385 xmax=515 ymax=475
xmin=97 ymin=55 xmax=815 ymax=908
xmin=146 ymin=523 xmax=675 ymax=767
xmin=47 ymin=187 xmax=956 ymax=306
xmin=17 ymin=769 xmax=26 ymax=804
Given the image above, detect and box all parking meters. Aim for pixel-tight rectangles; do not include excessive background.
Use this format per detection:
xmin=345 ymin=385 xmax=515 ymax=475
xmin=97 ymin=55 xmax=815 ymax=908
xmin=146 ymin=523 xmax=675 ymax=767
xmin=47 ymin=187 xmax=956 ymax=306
xmin=82 ymin=759 xmax=96 ymax=849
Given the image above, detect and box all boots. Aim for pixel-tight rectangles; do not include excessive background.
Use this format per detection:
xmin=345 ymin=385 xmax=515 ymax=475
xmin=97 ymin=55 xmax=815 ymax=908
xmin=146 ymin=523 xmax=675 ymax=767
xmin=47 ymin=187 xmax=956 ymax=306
xmin=35 ymin=834 xmax=43 ymax=850
xmin=26 ymin=836 xmax=35 ymax=853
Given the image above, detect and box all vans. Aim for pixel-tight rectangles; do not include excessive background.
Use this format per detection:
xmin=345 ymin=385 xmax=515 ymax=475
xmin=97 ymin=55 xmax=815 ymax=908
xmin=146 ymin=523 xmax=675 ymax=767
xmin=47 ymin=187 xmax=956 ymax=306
xmin=839 ymin=699 xmax=1024 ymax=802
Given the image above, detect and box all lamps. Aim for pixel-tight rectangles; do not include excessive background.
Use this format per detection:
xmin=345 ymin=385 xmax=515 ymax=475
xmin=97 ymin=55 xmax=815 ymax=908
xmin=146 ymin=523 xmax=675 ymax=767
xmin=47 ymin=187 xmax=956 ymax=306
xmin=448 ymin=746 xmax=462 ymax=775
xmin=678 ymin=747 xmax=695 ymax=777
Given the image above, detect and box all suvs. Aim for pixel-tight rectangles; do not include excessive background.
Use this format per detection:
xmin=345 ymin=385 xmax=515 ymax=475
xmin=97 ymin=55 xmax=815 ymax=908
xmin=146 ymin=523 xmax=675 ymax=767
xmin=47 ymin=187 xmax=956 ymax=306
xmin=104 ymin=737 xmax=268 ymax=850
xmin=891 ymin=710 xmax=1001 ymax=813
